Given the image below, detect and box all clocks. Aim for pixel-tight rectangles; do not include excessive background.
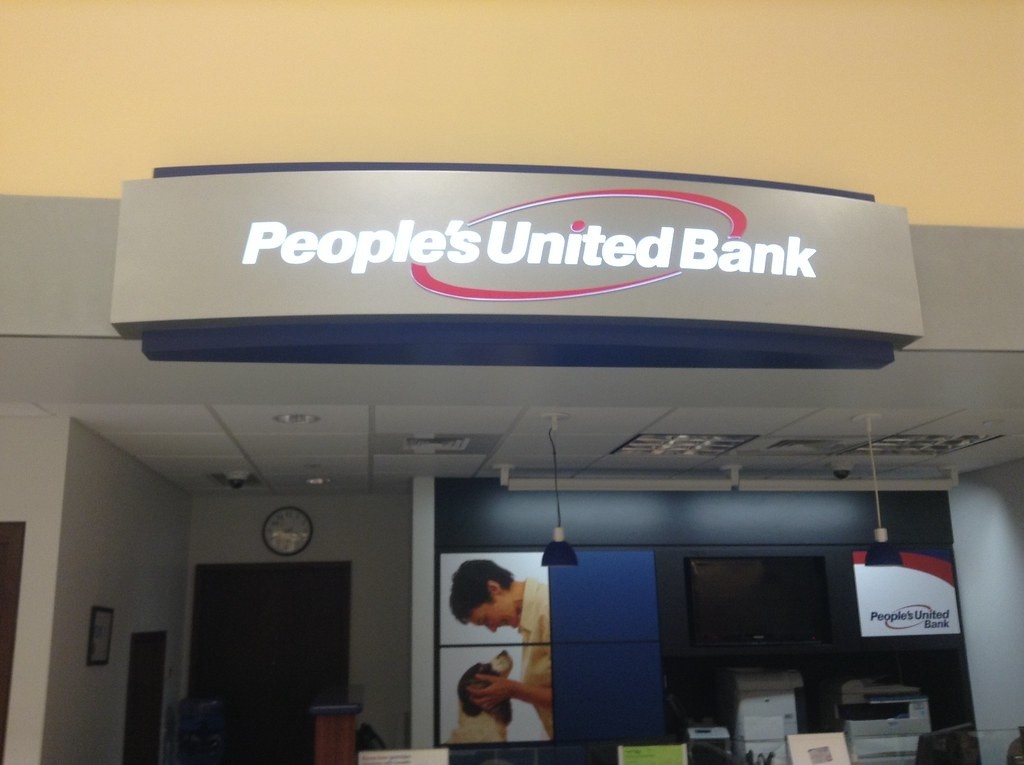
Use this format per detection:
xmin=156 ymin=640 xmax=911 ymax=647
xmin=262 ymin=506 xmax=313 ymax=556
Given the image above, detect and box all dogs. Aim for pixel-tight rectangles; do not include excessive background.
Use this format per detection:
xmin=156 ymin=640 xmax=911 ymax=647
xmin=445 ymin=650 xmax=514 ymax=743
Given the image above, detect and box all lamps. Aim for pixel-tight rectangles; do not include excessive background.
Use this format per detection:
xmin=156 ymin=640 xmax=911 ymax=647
xmin=542 ymin=413 xmax=578 ymax=566
xmin=851 ymin=414 xmax=902 ymax=568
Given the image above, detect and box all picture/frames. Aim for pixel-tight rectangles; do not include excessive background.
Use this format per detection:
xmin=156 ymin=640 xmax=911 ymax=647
xmin=86 ymin=606 xmax=115 ymax=665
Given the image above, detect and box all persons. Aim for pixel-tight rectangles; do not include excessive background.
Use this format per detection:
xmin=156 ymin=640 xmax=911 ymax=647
xmin=449 ymin=559 xmax=553 ymax=739
